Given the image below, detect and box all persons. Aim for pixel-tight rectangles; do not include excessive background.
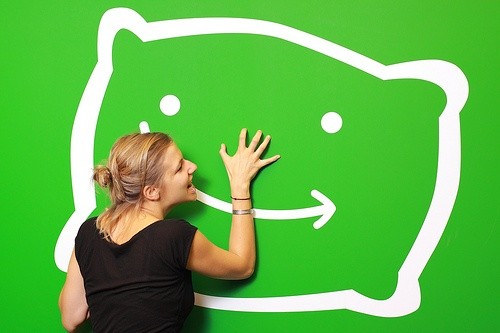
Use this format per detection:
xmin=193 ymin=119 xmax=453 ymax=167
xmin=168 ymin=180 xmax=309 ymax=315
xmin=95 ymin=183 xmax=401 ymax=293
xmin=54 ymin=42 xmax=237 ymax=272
xmin=58 ymin=126 xmax=281 ymax=333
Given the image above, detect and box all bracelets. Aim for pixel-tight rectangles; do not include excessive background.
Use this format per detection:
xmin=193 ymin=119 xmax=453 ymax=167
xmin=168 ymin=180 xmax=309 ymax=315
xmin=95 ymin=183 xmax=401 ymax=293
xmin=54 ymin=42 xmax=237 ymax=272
xmin=232 ymin=209 xmax=253 ymax=215
xmin=230 ymin=195 xmax=251 ymax=200
